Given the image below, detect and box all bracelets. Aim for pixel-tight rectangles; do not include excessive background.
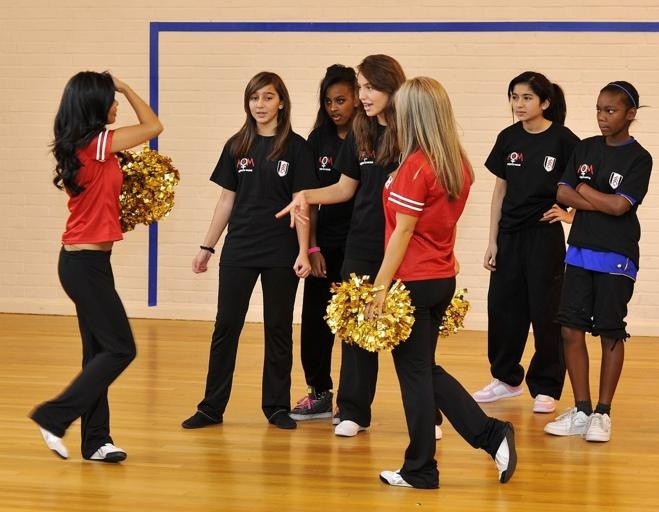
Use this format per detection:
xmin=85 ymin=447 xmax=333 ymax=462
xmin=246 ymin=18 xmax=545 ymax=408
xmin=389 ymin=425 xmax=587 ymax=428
xmin=306 ymin=246 xmax=321 ymax=256
xmin=198 ymin=245 xmax=215 ymax=254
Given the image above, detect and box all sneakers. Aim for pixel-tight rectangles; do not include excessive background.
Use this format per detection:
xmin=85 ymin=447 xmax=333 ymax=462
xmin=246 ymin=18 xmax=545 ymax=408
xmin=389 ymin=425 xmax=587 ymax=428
xmin=87 ymin=442 xmax=128 ymax=464
xmin=435 ymin=424 xmax=442 ymax=440
xmin=494 ymin=421 xmax=518 ymax=485
xmin=181 ymin=410 xmax=224 ymax=429
xmin=288 ymin=385 xmax=333 ymax=421
xmin=269 ymin=409 xmax=297 ymax=429
xmin=333 ymin=409 xmax=371 ymax=437
xmin=37 ymin=423 xmax=71 ymax=462
xmin=379 ymin=468 xmax=417 ymax=489
xmin=533 ymin=393 xmax=557 ymax=414
xmin=471 ymin=377 xmax=524 ymax=404
xmin=543 ymin=406 xmax=594 ymax=436
xmin=583 ymin=411 xmax=611 ymax=443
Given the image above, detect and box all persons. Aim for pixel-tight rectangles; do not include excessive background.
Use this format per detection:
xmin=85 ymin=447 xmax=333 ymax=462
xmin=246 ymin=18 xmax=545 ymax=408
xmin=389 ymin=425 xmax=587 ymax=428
xmin=362 ymin=78 xmax=517 ymax=487
xmin=275 ymin=54 xmax=459 ymax=438
xmin=541 ymin=80 xmax=653 ymax=445
xmin=472 ymin=70 xmax=582 ymax=414
xmin=286 ymin=64 xmax=363 ymax=422
xmin=24 ymin=68 xmax=165 ymax=465
xmin=179 ymin=70 xmax=312 ymax=430
